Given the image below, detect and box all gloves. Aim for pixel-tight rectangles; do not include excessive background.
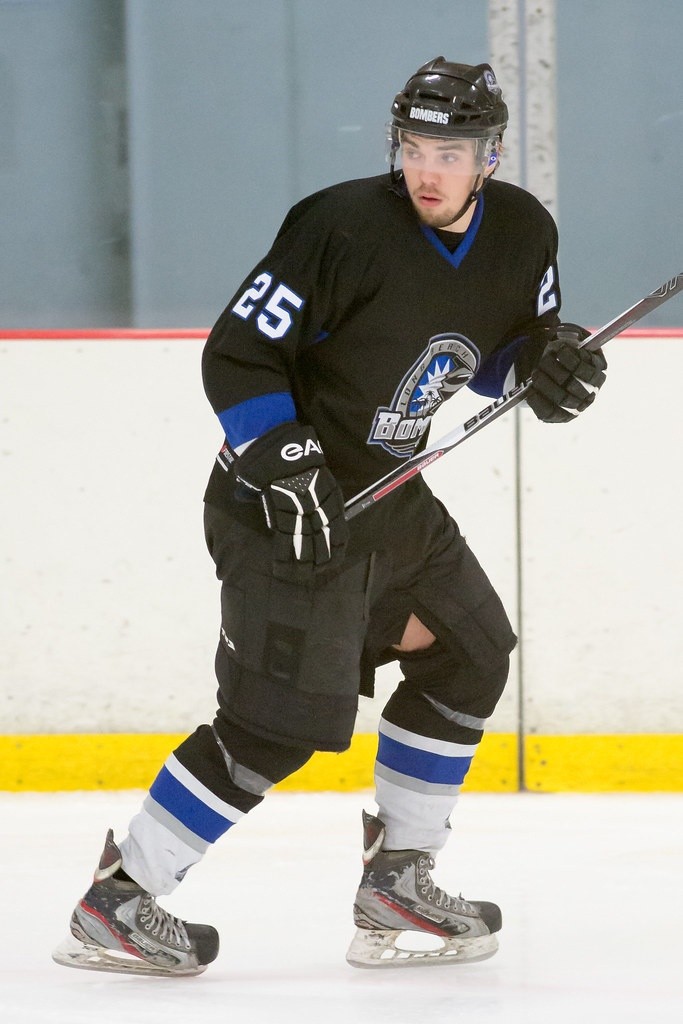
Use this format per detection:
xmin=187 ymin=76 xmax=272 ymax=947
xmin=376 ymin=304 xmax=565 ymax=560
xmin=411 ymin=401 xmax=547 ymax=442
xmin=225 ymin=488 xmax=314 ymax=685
xmin=513 ymin=322 xmax=607 ymax=424
xmin=229 ymin=422 xmax=349 ymax=566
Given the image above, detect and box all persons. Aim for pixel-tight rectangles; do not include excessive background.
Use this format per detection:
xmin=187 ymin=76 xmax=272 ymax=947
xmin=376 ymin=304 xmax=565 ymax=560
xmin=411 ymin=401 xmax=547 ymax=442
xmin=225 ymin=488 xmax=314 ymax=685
xmin=52 ymin=56 xmax=606 ymax=978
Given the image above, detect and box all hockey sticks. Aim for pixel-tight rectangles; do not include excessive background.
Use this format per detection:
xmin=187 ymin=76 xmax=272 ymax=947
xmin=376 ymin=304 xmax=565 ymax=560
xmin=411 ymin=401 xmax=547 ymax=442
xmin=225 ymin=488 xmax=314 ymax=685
xmin=338 ymin=270 xmax=683 ymax=525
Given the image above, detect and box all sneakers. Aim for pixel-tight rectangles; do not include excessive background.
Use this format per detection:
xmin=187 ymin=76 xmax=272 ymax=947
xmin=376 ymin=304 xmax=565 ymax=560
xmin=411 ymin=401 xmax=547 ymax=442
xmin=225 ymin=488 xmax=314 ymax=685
xmin=52 ymin=828 xmax=220 ymax=978
xmin=345 ymin=809 xmax=502 ymax=969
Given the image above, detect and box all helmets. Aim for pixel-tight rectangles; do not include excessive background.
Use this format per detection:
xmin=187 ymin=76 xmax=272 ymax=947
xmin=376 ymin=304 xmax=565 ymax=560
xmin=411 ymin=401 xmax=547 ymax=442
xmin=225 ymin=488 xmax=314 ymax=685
xmin=388 ymin=55 xmax=509 ymax=139
